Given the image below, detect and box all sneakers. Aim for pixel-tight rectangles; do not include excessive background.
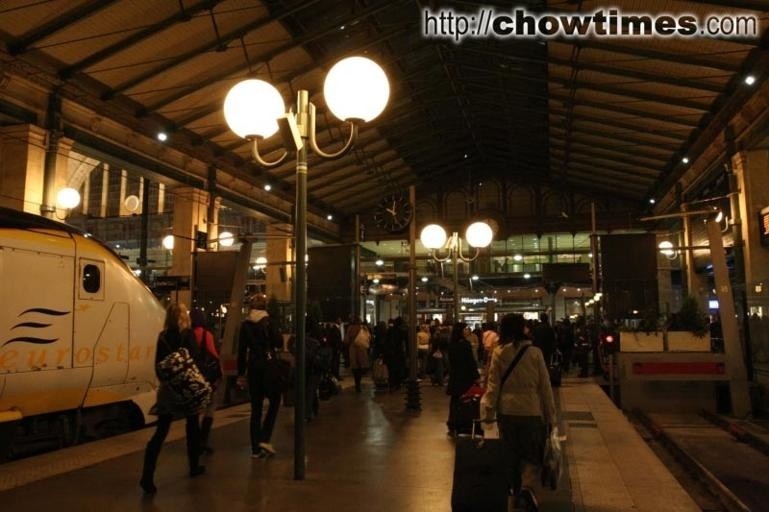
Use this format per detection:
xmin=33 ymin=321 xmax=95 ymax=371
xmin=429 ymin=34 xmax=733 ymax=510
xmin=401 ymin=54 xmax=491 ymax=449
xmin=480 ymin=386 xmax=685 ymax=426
xmin=251 ymin=449 xmax=266 ymax=459
xmin=259 ymin=442 xmax=276 ymax=455
xmin=523 ymin=487 xmax=538 ymax=510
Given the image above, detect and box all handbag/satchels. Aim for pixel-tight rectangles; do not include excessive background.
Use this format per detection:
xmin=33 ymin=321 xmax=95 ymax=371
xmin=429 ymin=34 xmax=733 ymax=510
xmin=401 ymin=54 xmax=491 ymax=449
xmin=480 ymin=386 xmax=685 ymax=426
xmin=433 ymin=351 xmax=443 ymax=358
xmin=156 ymin=348 xmax=211 ymax=418
xmin=459 ymin=384 xmax=483 ymax=406
xmin=354 ymin=328 xmax=370 ymax=350
xmin=318 ymin=375 xmax=340 ymax=400
xmin=254 ymin=346 xmax=292 ymax=377
xmin=197 ymin=348 xmax=220 ymax=383
xmin=543 ymin=426 xmax=561 ymax=491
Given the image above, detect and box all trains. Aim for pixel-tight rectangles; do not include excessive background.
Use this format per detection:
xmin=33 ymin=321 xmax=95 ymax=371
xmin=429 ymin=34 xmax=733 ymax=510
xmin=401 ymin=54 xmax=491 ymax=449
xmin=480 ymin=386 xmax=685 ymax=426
xmin=1 ymin=204 xmax=167 ymax=464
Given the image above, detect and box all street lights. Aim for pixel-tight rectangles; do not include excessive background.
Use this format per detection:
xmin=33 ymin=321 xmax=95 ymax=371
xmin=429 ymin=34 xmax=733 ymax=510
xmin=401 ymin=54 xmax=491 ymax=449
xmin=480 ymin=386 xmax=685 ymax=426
xmin=419 ymin=219 xmax=495 ymax=326
xmin=223 ymin=55 xmax=391 ymax=483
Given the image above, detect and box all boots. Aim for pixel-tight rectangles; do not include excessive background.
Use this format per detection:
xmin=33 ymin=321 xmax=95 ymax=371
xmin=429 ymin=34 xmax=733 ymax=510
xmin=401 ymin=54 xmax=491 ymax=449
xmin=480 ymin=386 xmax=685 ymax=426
xmin=186 ymin=424 xmax=205 ymax=477
xmin=141 ymin=448 xmax=157 ymax=493
xmin=200 ymin=417 xmax=213 ymax=455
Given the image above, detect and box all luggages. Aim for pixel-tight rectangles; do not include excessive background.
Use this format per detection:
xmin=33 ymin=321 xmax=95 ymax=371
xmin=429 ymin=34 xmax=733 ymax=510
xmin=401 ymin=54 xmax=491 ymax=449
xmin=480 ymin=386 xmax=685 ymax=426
xmin=373 ymin=358 xmax=390 ymax=386
xmin=456 ymin=382 xmax=483 ymax=436
xmin=547 ymin=347 xmax=561 ymax=387
xmin=451 ymin=419 xmax=511 ymax=511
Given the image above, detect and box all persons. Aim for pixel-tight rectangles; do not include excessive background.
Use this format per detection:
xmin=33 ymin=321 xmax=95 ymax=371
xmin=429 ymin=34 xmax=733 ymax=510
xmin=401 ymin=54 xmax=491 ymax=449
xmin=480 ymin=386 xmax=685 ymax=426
xmin=482 ymin=311 xmax=557 ymax=512
xmin=447 ymin=321 xmax=484 ymax=442
xmin=139 ymin=302 xmax=208 ymax=493
xmin=236 ymin=293 xmax=285 ymax=460
xmin=187 ymin=310 xmax=221 ymax=457
xmin=289 ymin=304 xmax=769 ymax=425
